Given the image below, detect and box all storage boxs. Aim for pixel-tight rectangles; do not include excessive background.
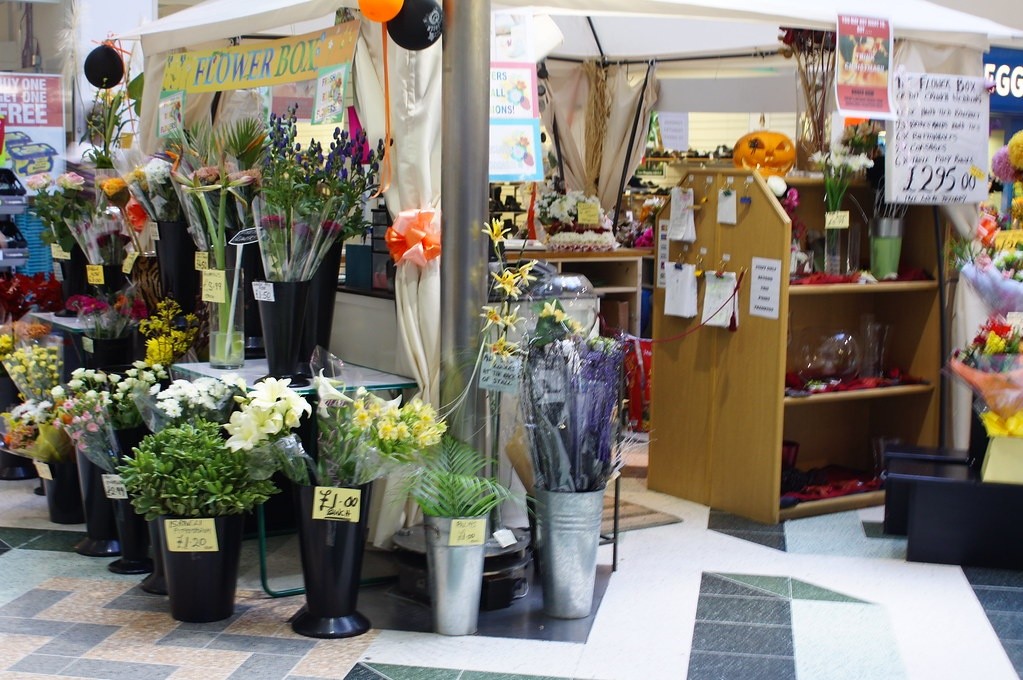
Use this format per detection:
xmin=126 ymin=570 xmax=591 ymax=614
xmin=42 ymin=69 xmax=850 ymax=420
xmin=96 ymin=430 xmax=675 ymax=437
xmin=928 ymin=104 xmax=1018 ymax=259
xmin=396 ymin=521 xmax=538 ymax=611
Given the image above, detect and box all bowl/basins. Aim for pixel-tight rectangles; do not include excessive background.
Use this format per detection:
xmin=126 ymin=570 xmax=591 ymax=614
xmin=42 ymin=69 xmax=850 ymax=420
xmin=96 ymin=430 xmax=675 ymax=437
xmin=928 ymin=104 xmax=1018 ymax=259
xmin=795 ymin=326 xmax=860 ymax=388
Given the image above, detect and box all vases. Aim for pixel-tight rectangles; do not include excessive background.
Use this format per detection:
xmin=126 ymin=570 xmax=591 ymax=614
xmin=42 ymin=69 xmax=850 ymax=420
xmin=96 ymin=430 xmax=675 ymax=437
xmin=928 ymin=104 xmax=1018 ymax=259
xmin=824 ymin=210 xmax=854 ymax=276
xmin=867 ymin=215 xmax=903 ymax=279
xmin=0 ymin=181 xmax=609 ymax=641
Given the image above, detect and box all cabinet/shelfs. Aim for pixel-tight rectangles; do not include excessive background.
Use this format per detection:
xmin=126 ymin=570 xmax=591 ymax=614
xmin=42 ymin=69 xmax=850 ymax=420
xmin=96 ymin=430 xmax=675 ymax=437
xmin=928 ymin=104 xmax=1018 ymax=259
xmin=653 ymin=164 xmax=952 ymax=526
xmin=500 ymin=243 xmax=653 ymax=340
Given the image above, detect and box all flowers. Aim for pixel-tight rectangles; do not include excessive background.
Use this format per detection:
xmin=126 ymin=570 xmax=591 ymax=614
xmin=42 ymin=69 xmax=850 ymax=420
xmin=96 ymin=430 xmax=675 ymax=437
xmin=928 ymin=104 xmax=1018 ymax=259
xmin=781 ymin=27 xmax=836 ymax=146
xmin=807 ymin=115 xmax=880 ymax=211
xmin=0 ymin=108 xmax=661 ymax=518
xmin=941 ymin=133 xmax=1023 ymax=483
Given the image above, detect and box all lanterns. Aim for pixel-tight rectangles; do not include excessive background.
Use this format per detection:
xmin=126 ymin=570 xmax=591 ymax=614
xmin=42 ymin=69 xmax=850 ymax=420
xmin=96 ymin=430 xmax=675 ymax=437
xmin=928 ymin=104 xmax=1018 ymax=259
xmin=732 ymin=131 xmax=796 ymax=172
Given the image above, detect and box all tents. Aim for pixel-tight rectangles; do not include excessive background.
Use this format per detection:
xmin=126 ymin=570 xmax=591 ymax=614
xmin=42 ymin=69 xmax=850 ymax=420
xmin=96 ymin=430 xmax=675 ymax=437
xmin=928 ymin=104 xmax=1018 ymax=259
xmin=135 ymin=0 xmax=1022 ymax=451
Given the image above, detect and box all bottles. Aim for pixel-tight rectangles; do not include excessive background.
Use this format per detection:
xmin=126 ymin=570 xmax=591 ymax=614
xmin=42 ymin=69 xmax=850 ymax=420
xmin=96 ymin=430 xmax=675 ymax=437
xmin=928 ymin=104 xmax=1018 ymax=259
xmin=849 ymin=309 xmax=879 ymax=377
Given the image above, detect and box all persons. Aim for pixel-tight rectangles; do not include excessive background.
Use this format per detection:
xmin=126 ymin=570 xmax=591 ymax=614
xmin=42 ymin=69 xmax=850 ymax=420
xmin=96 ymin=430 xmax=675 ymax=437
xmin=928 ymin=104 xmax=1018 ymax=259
xmin=845 ymin=34 xmax=887 ymax=84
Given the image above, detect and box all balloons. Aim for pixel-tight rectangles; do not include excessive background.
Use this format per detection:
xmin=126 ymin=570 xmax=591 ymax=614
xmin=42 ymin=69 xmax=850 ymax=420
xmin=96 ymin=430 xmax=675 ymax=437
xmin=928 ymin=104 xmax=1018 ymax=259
xmin=358 ymin=0 xmax=404 ymax=22
xmin=387 ymin=0 xmax=442 ymax=51
